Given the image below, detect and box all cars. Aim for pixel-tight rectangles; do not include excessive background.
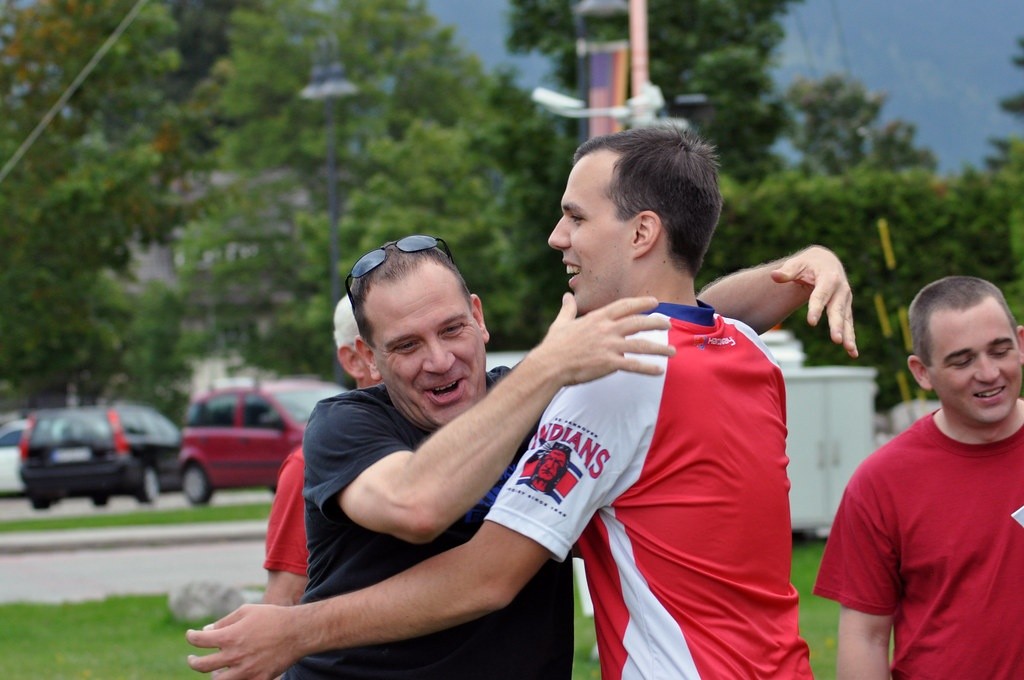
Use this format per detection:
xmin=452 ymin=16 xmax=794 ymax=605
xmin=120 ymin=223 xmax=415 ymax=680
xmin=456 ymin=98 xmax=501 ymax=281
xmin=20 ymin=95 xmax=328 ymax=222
xmin=0 ymin=416 xmax=30 ymax=497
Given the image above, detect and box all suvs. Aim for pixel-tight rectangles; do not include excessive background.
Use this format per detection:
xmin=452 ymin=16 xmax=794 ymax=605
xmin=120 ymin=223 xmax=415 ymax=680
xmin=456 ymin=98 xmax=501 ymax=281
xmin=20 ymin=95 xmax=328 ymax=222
xmin=180 ymin=376 xmax=352 ymax=507
xmin=18 ymin=401 xmax=183 ymax=508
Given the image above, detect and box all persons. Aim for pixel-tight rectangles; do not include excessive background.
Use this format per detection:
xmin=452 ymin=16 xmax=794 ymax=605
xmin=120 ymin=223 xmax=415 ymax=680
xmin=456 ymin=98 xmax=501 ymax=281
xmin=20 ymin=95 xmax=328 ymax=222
xmin=272 ymin=236 xmax=858 ymax=680
xmin=812 ymin=273 xmax=1024 ymax=680
xmin=254 ymin=289 xmax=390 ymax=646
xmin=183 ymin=121 xmax=814 ymax=680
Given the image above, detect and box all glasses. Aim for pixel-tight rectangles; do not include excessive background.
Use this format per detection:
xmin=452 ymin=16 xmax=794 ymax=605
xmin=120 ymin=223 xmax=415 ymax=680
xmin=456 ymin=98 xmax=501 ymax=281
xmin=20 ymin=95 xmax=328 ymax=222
xmin=346 ymin=234 xmax=454 ymax=311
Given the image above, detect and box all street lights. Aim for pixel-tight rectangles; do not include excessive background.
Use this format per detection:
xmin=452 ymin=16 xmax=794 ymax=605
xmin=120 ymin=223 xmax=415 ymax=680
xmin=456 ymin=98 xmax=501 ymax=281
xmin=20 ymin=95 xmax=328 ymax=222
xmin=299 ymin=35 xmax=358 ymax=389
